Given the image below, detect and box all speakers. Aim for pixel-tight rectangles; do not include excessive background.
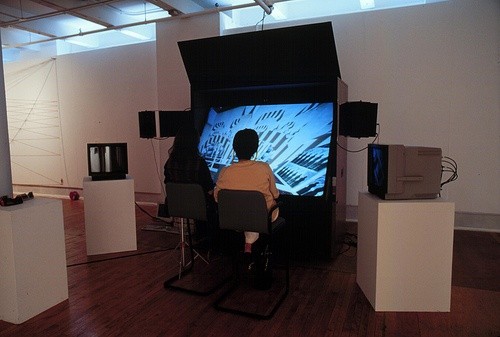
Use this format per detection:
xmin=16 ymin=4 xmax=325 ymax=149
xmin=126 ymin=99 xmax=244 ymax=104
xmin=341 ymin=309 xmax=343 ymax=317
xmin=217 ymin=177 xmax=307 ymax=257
xmin=138 ymin=111 xmax=156 ymax=139
xmin=339 ymin=101 xmax=378 ymax=138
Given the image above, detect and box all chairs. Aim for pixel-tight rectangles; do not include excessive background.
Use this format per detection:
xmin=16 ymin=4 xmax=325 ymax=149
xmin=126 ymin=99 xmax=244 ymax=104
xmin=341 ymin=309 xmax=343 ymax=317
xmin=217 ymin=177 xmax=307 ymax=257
xmin=163 ymin=183 xmax=228 ymax=297
xmin=212 ymin=189 xmax=291 ymax=320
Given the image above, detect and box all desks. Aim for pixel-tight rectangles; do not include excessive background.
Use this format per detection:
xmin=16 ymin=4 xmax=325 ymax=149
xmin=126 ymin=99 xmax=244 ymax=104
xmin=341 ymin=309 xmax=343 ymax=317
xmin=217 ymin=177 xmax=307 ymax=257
xmin=82 ymin=176 xmax=137 ymax=255
xmin=356 ymin=191 xmax=454 ymax=312
xmin=1 ymin=198 xmax=69 ymax=325
